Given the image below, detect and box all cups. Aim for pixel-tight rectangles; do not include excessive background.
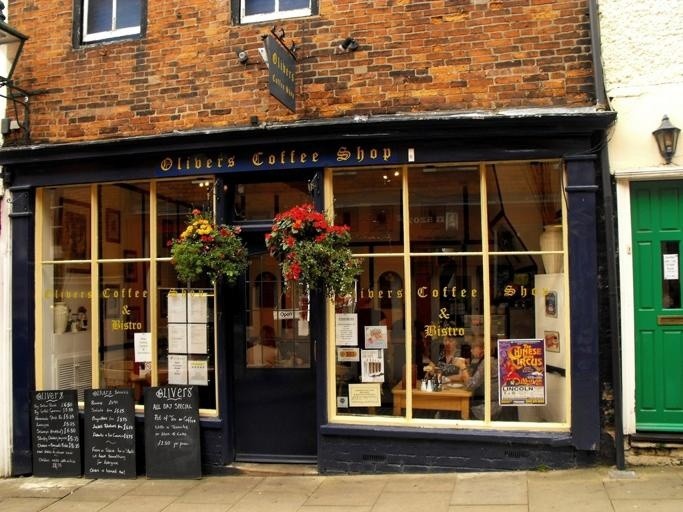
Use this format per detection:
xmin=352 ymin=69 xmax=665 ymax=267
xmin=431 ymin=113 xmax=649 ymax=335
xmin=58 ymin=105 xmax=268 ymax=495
xmin=454 ymin=357 xmax=466 ymax=365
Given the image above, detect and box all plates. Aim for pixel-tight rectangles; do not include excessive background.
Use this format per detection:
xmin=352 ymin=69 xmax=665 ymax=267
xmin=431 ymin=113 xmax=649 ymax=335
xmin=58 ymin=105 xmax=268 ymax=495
xmin=447 ymin=383 xmax=464 ymax=389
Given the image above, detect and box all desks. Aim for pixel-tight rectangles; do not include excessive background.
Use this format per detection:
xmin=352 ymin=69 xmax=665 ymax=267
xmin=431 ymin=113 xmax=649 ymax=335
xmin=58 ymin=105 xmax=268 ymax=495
xmin=392 ymin=376 xmax=473 ymax=420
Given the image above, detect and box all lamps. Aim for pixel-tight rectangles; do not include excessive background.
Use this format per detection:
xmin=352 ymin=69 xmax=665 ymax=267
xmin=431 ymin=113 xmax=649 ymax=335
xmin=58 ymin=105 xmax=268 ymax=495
xmin=338 ymin=38 xmax=359 ymax=53
xmin=650 ymin=113 xmax=681 ymax=166
xmin=235 ymin=49 xmax=249 ymax=67
xmin=0 ymin=0 xmax=33 ymax=146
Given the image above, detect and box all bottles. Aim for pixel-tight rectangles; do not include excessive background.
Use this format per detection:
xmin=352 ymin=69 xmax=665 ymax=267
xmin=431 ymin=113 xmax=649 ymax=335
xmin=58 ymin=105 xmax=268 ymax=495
xmin=420 ymin=380 xmax=433 ymax=392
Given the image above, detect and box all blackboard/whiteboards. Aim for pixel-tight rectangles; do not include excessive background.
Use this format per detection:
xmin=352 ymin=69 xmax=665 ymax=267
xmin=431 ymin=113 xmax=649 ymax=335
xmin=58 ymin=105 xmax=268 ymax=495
xmin=144 ymin=386 xmax=203 ymax=480
xmin=30 ymin=390 xmax=82 ymax=477
xmin=83 ymin=387 xmax=137 ymax=479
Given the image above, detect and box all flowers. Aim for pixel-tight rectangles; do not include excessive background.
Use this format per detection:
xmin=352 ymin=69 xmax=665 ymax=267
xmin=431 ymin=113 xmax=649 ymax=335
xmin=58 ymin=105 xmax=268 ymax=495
xmin=167 ymin=209 xmax=246 ymax=285
xmin=264 ymin=206 xmax=359 ymax=296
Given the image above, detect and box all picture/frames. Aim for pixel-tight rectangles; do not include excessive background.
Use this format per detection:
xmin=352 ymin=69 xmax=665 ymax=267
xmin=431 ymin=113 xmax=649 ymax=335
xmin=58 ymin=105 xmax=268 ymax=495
xmin=55 ymin=196 xmax=91 ymax=274
xmin=103 ymin=207 xmax=142 ymax=339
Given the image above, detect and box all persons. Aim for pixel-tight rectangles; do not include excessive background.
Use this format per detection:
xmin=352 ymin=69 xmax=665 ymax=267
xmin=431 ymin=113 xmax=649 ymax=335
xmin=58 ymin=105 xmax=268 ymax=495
xmin=392 ymin=320 xmax=504 ymax=420
xmin=76 ymin=305 xmax=88 ymax=332
xmin=247 ymin=325 xmax=309 ymax=368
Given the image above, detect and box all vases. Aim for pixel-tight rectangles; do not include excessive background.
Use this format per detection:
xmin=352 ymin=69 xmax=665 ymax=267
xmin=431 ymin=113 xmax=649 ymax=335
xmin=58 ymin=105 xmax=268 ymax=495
xmin=538 ymin=223 xmax=565 ymax=273
